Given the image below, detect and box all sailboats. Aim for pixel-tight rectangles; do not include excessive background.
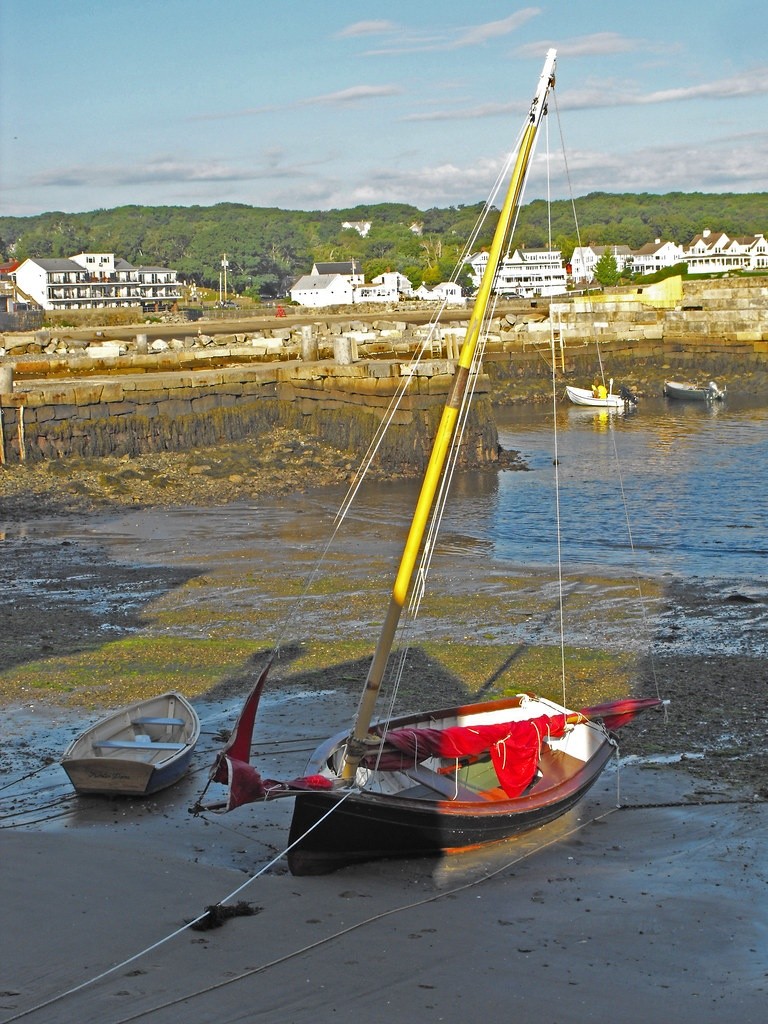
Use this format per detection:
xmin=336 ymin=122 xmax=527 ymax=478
xmin=182 ymin=48 xmax=675 ymax=879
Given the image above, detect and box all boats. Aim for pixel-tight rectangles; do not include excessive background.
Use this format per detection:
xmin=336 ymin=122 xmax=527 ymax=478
xmin=663 ymin=378 xmax=729 ymax=400
xmin=564 ymin=378 xmax=641 ymax=406
xmin=59 ymin=691 xmax=203 ymax=800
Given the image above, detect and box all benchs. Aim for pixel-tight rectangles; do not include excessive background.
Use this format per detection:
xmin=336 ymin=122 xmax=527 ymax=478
xmin=93 ymin=740 xmax=184 ymax=750
xmin=131 ymin=716 xmax=184 ymax=726
xmin=481 ymin=740 xmax=586 ymax=795
xmin=399 ymin=763 xmax=486 ymax=802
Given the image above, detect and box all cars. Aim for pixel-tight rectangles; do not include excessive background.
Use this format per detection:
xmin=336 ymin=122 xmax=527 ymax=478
xmin=502 ymin=292 xmax=524 ymax=300
xmin=222 ymin=301 xmax=239 ymax=308
